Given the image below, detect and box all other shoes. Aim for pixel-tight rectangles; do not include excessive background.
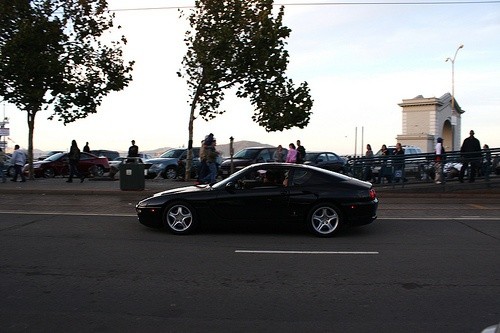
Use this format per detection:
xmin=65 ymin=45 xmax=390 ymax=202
xmin=11 ymin=179 xmax=16 ymax=182
xmin=436 ymin=181 xmax=441 ymax=184
xmin=20 ymin=180 xmax=26 ymax=182
xmin=81 ymin=178 xmax=85 ymax=183
xmin=66 ymin=180 xmax=72 ymax=182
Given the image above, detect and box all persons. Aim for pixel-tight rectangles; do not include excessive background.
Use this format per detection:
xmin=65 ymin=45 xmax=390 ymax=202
xmin=66 ymin=140 xmax=85 ymax=183
xmin=197 ymin=133 xmax=221 ymax=183
xmin=433 ymin=138 xmax=446 ymax=184
xmin=10 ymin=145 xmax=27 ymax=182
xmin=238 ymin=170 xmax=268 ymax=188
xmin=296 ymin=140 xmax=306 ymax=163
xmin=458 ymin=129 xmax=482 ymax=182
xmin=127 ymin=140 xmax=139 ymax=157
xmin=365 ymin=143 xmax=374 ymax=180
xmin=392 ymin=143 xmax=406 ymax=183
xmin=483 ymin=144 xmax=492 ymax=179
xmin=376 ymin=145 xmax=391 ymax=183
xmin=286 ymin=142 xmax=299 ymax=163
xmin=0 ymin=148 xmax=6 ymax=182
xmin=272 ymin=144 xmax=287 ymax=162
xmin=83 ymin=142 xmax=91 ymax=153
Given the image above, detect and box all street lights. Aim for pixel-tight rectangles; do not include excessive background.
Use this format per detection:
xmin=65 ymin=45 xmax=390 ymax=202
xmin=446 ymin=44 xmax=465 ymax=177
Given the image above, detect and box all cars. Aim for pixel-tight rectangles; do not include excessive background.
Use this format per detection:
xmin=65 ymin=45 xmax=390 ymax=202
xmin=0 ymin=153 xmax=16 ymax=178
xmin=22 ymin=149 xmax=153 ymax=178
xmin=304 ymin=151 xmax=349 ymax=173
xmin=136 ymin=162 xmax=379 ymax=239
xmin=443 ymin=152 xmax=500 ymax=176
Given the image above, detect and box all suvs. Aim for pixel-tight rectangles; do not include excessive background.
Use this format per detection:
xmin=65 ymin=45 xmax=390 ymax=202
xmin=218 ymin=147 xmax=288 ymax=179
xmin=141 ymin=147 xmax=222 ymax=180
xmin=358 ymin=145 xmax=433 ymax=182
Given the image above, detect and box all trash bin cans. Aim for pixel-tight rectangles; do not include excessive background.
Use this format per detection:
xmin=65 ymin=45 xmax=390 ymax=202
xmin=119 ymin=156 xmax=153 ymax=191
